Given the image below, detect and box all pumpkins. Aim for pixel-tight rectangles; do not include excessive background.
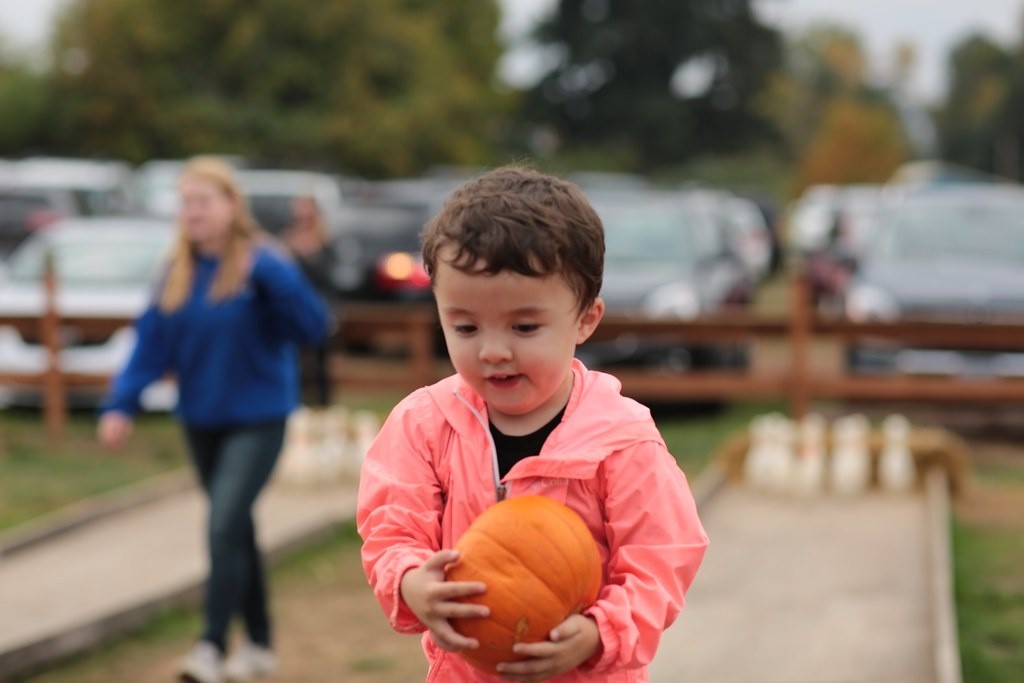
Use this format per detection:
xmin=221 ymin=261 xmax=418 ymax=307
xmin=442 ymin=493 xmax=603 ymax=674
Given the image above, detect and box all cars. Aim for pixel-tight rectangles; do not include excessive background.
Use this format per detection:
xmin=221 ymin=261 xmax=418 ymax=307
xmin=846 ymin=189 xmax=1024 ymax=376
xmin=2 ymin=157 xmax=769 ymax=424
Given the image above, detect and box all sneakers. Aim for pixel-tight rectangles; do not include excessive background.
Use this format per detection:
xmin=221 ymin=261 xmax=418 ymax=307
xmin=172 ymin=638 xmax=225 ymax=683
xmin=224 ymin=641 xmax=278 ymax=681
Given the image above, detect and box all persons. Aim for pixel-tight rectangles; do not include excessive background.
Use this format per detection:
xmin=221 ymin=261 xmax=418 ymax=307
xmin=284 ymin=194 xmax=335 ymax=407
xmin=809 ymin=206 xmax=863 ymax=312
xmin=356 ymin=165 xmax=708 ymax=683
xmin=98 ymin=159 xmax=332 ymax=683
